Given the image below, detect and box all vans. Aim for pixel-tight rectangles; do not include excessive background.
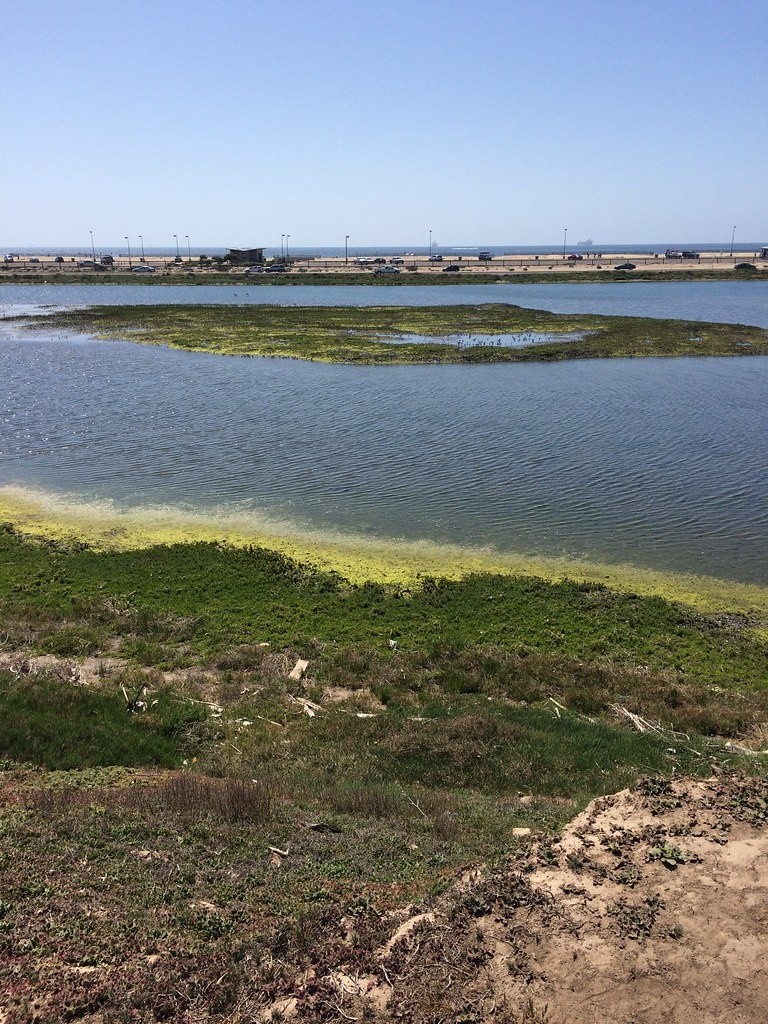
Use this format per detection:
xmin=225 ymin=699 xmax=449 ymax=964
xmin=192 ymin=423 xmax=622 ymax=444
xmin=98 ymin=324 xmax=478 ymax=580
xmin=4 ymin=255 xmax=13 ymax=263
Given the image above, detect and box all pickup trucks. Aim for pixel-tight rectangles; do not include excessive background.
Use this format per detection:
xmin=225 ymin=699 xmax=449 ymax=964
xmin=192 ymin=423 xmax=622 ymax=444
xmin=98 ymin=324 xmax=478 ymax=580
xmin=77 ymin=260 xmax=98 ymax=268
xmin=100 ymin=256 xmax=113 ymax=265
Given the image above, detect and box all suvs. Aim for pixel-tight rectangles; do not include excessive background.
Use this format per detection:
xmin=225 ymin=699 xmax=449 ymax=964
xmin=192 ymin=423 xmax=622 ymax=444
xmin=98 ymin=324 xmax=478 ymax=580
xmin=442 ymin=264 xmax=460 ymax=272
xmin=429 ymin=254 xmax=442 ymax=262
xmin=682 ymin=250 xmax=700 ymax=259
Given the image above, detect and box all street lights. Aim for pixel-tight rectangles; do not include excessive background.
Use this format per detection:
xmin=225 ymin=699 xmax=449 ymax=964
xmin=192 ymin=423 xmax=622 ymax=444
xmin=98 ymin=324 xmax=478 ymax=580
xmin=173 ymin=235 xmax=179 ymax=259
xmin=346 ymin=236 xmax=350 ymax=265
xmin=286 ymin=235 xmax=291 ymax=259
xmin=282 ymin=234 xmax=285 ymax=257
xmin=185 ymin=235 xmax=191 ymax=260
xmin=89 ymin=230 xmax=95 ymax=262
xmin=138 ymin=235 xmax=144 ymax=258
xmin=729 ymin=226 xmax=737 ymax=256
xmin=125 ymin=237 xmax=131 ymax=261
xmin=563 ymin=228 xmax=567 ymax=259
xmin=430 ymin=230 xmax=432 ymax=257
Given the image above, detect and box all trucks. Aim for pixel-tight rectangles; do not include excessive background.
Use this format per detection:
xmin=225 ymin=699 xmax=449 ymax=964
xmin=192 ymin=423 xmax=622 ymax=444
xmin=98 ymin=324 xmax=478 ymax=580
xmin=479 ymin=250 xmax=495 ymax=262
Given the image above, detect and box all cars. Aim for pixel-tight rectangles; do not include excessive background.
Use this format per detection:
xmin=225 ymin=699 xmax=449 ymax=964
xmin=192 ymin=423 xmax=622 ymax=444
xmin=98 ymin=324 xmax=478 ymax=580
xmin=55 ymin=256 xmax=64 ymax=262
xmin=132 ymin=265 xmax=155 ymax=272
xmin=733 ymin=263 xmax=757 ymax=271
xmin=568 ymin=253 xmax=584 ymax=261
xmin=245 ymin=264 xmax=292 ymax=273
xmin=29 ymin=256 xmax=39 ymax=263
xmin=353 ymin=256 xmax=405 ymax=274
xmin=613 ymin=263 xmax=637 ymax=271
